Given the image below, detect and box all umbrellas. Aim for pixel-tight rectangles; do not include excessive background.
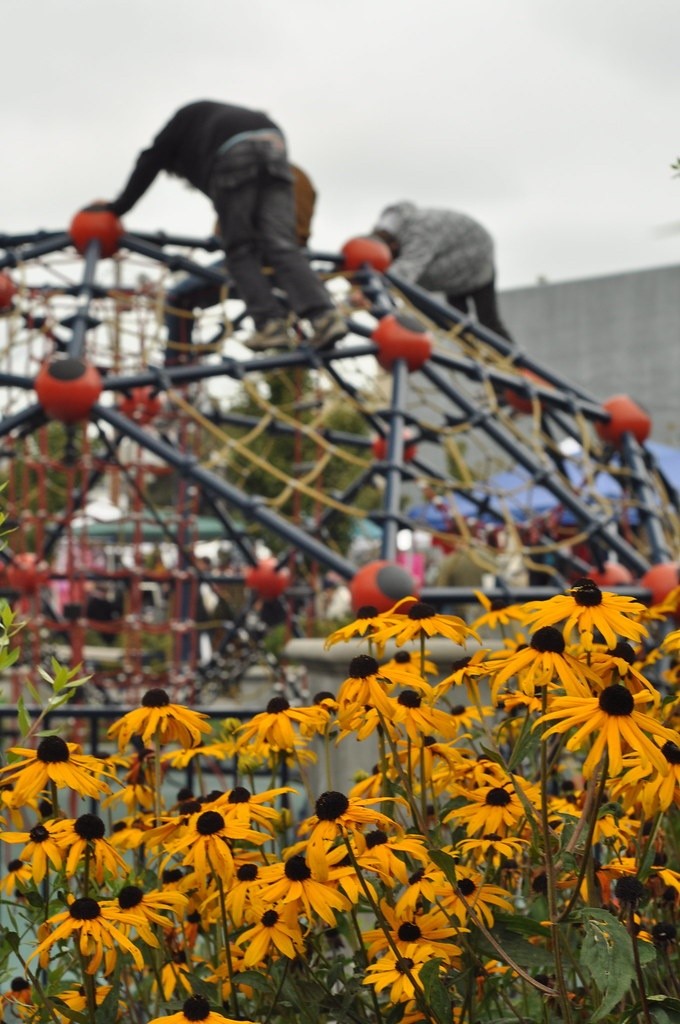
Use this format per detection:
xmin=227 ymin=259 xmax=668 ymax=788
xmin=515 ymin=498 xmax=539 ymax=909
xmin=70 ymin=505 xmax=247 ymax=548
xmin=411 ymin=425 xmax=680 ymax=527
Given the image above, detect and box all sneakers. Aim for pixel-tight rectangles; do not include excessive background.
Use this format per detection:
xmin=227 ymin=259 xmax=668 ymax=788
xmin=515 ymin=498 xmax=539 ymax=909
xmin=243 ymin=320 xmax=291 ymax=351
xmin=312 ymin=310 xmax=348 ymax=350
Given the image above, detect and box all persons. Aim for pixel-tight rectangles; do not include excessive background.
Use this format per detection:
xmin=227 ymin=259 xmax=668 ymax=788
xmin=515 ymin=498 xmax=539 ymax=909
xmin=87 ymin=555 xmax=288 ymax=704
xmin=433 ymin=519 xmax=572 ymax=587
xmin=82 ymin=100 xmax=348 ymax=352
xmin=335 ymin=201 xmax=527 ymax=406
xmin=165 ymin=159 xmax=316 ymax=371
